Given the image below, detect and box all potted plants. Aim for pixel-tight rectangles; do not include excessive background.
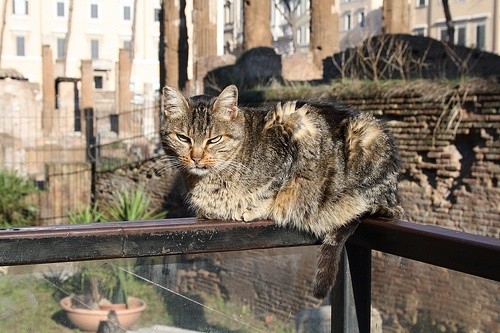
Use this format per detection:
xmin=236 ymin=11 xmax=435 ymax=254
xmin=59 ymin=189 xmax=168 ymax=331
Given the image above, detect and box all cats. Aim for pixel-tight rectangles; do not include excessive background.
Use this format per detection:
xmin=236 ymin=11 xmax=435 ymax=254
xmin=159 ymin=85 xmax=404 ymax=302
